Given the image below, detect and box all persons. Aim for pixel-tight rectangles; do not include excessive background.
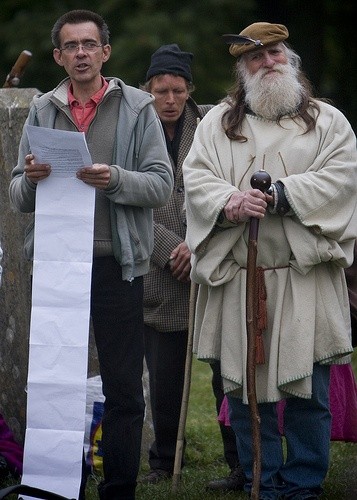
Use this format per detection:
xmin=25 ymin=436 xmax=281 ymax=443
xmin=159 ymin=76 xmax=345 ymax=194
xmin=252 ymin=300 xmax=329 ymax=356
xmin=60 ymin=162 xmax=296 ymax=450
xmin=138 ymin=43 xmax=240 ymax=492
xmin=181 ymin=21 xmax=357 ymax=500
xmin=9 ymin=9 xmax=174 ymax=500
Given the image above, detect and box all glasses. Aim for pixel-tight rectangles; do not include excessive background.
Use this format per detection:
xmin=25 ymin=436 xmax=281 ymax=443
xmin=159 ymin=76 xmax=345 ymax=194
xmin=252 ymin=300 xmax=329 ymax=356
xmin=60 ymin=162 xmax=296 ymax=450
xmin=59 ymin=42 xmax=102 ymax=55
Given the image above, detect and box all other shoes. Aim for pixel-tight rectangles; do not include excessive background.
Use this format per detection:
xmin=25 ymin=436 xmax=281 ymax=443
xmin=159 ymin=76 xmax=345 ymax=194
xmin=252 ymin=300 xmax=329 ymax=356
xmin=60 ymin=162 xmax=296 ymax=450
xmin=205 ymin=468 xmax=246 ymax=492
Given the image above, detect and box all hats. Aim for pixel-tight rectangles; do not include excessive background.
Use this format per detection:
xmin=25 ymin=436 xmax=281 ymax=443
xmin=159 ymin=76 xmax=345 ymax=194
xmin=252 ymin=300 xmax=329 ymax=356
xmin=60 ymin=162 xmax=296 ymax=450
xmin=146 ymin=43 xmax=193 ymax=81
xmin=221 ymin=22 xmax=289 ymax=57
